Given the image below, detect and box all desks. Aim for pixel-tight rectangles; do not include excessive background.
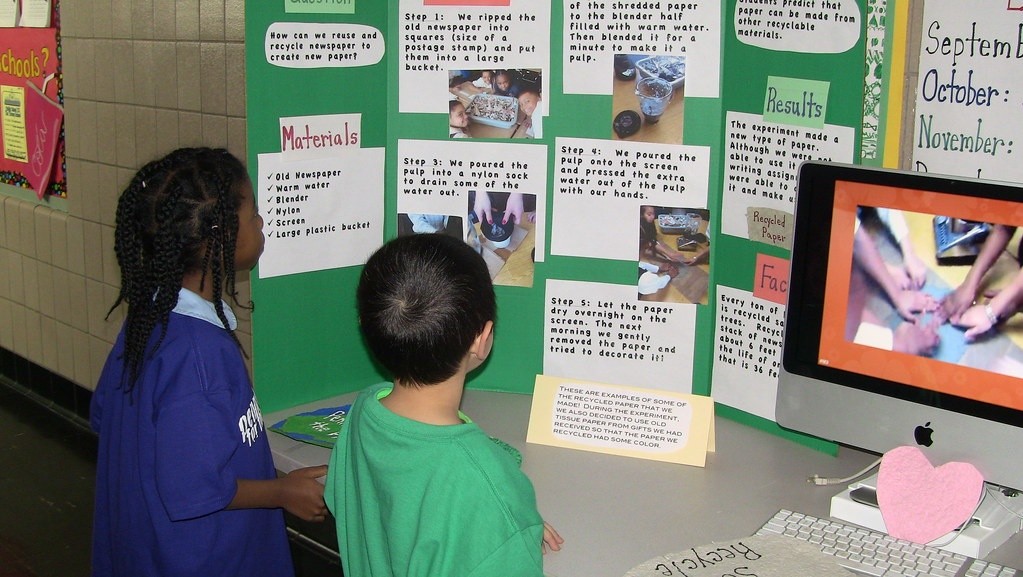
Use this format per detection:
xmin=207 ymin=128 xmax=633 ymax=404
xmin=262 ymin=390 xmax=1023 ymax=577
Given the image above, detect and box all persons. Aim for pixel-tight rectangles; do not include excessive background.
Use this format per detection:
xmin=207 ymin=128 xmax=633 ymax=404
xmin=407 ymin=213 xmax=452 ymax=234
xmin=449 ymin=70 xmax=544 ymax=139
xmin=637 ymin=206 xmax=710 ymax=301
xmin=89 ymin=146 xmax=329 ymax=577
xmin=852 ymin=204 xmax=1023 ymax=357
xmin=321 ymin=232 xmax=564 ymax=577
xmin=474 ymin=191 xmax=537 ymax=226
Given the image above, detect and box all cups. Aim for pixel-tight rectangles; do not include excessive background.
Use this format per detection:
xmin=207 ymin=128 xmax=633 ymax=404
xmin=687 ymin=213 xmax=702 ymax=235
xmin=481 ymin=213 xmax=514 ymax=248
xmin=635 ymin=78 xmax=672 ymax=123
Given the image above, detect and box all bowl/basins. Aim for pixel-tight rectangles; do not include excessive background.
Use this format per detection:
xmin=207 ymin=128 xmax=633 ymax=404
xmin=659 ymin=215 xmax=687 ymax=234
xmin=469 ymin=94 xmax=518 ymax=127
xmin=635 ymin=55 xmax=685 ymax=89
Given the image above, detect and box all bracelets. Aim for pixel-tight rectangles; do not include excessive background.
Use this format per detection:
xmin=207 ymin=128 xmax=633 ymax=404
xmin=985 ymin=304 xmax=997 ymax=325
xmin=693 ymin=256 xmax=698 ymax=261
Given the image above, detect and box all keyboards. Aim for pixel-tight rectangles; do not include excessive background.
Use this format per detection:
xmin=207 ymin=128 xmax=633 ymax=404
xmin=748 ymin=508 xmax=1023 ymax=577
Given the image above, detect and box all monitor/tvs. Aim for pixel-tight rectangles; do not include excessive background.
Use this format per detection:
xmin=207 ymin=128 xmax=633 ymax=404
xmin=774 ymin=161 xmax=1023 ymax=562
